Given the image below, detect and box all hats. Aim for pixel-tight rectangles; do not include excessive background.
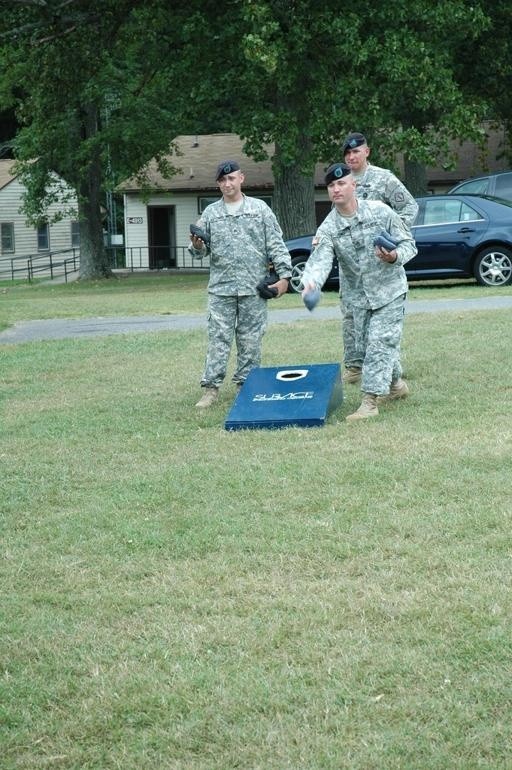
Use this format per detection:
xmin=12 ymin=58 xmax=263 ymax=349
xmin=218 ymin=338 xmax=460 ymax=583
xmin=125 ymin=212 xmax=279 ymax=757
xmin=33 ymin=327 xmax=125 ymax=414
xmin=215 ymin=161 xmax=241 ymax=182
xmin=343 ymin=133 xmax=366 ymax=156
xmin=325 ymin=163 xmax=351 ymax=187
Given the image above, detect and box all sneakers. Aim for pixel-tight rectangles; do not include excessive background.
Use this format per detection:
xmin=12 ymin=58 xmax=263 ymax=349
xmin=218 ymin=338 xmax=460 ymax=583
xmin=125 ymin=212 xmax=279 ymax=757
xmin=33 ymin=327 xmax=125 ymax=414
xmin=343 ymin=367 xmax=363 ymax=384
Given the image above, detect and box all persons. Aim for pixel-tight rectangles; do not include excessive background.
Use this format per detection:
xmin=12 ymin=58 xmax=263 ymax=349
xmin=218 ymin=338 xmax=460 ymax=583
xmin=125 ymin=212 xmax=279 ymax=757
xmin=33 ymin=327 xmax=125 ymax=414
xmin=324 ymin=131 xmax=421 ymax=384
xmin=187 ymin=160 xmax=294 ymax=409
xmin=298 ymin=162 xmax=419 ymax=422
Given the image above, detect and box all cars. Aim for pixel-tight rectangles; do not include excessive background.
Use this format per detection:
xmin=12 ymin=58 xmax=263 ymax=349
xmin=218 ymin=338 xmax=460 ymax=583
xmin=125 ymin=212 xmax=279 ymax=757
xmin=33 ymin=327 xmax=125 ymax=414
xmin=266 ymin=193 xmax=512 ymax=294
xmin=446 ymin=171 xmax=512 ymax=203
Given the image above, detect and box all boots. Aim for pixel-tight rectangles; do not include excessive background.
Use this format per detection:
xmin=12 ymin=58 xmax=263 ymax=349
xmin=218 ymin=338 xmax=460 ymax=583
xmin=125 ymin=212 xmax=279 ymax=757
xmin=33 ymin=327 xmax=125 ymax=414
xmin=196 ymin=387 xmax=219 ymax=409
xmin=377 ymin=378 xmax=412 ymax=402
xmin=346 ymin=391 xmax=380 ymax=422
